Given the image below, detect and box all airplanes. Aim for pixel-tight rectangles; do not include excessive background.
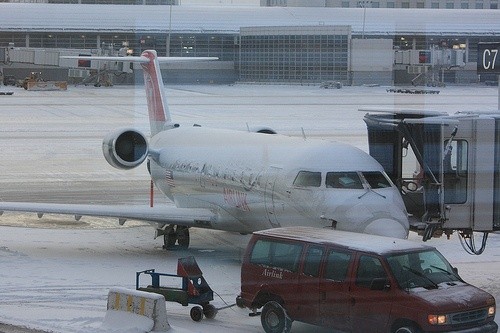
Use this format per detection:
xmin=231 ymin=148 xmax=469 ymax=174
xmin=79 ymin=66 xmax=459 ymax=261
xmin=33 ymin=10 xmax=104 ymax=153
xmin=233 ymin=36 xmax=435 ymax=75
xmin=0 ymin=48 xmax=411 ymax=250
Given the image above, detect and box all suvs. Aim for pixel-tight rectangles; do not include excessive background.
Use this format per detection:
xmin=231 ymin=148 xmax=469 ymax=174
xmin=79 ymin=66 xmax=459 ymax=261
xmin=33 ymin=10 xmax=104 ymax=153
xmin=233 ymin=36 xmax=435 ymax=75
xmin=235 ymin=225 xmax=500 ymax=333
